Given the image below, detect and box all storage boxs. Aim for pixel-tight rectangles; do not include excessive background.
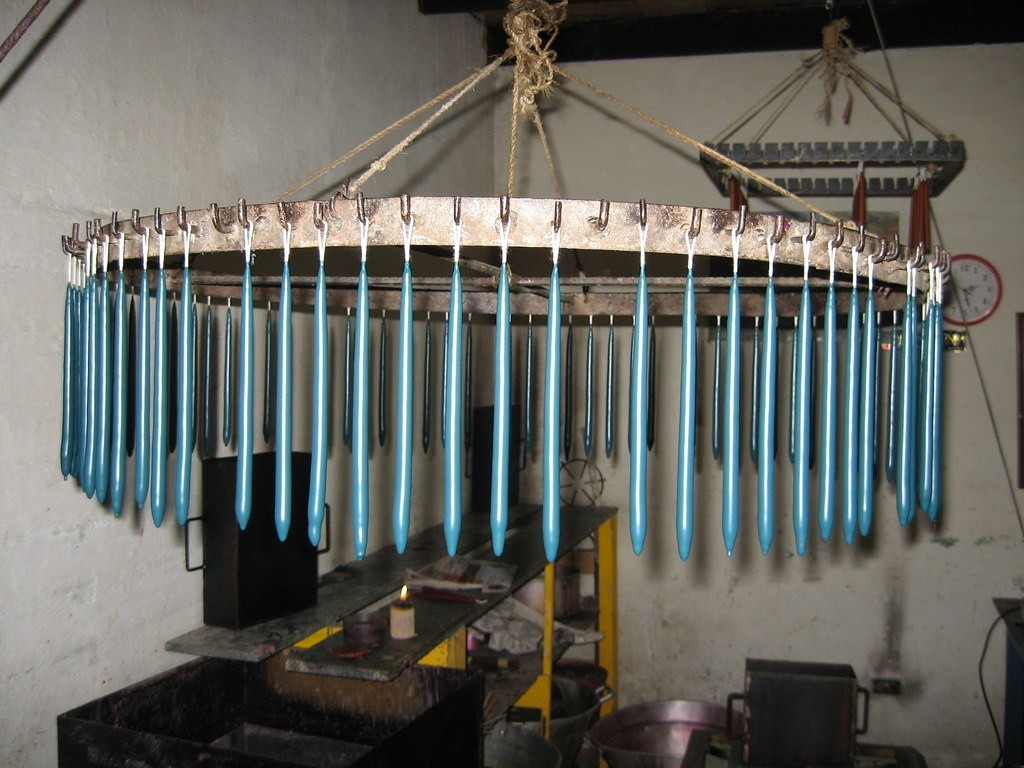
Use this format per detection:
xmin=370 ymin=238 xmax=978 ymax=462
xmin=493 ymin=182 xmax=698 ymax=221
xmin=185 ymin=450 xmax=331 ymax=631
xmin=57 ymin=656 xmax=484 ymax=768
xmin=465 ymin=405 xmax=527 ymax=513
xmin=725 ymin=657 xmax=870 ymax=768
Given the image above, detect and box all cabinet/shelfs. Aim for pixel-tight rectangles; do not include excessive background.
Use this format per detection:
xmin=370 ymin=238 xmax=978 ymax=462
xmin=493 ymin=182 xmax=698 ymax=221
xmin=161 ymin=504 xmax=620 ymax=768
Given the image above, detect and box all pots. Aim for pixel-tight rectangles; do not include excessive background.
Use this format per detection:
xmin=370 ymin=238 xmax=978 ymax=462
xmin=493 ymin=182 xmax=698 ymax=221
xmin=518 ymin=675 xmax=613 ymax=768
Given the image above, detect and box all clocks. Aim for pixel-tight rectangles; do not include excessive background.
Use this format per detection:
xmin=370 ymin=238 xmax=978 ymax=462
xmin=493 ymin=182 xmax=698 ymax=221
xmin=942 ymin=254 xmax=1002 ymax=327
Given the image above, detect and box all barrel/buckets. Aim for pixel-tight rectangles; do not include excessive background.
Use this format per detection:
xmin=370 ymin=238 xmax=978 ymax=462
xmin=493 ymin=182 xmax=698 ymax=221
xmin=592 ymin=700 xmax=749 ymax=768
xmin=483 ymin=721 xmax=561 ymax=768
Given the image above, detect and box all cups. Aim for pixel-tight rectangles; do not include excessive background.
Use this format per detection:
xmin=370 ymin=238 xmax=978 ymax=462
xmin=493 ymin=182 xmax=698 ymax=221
xmin=343 ymin=614 xmax=387 ymax=650
